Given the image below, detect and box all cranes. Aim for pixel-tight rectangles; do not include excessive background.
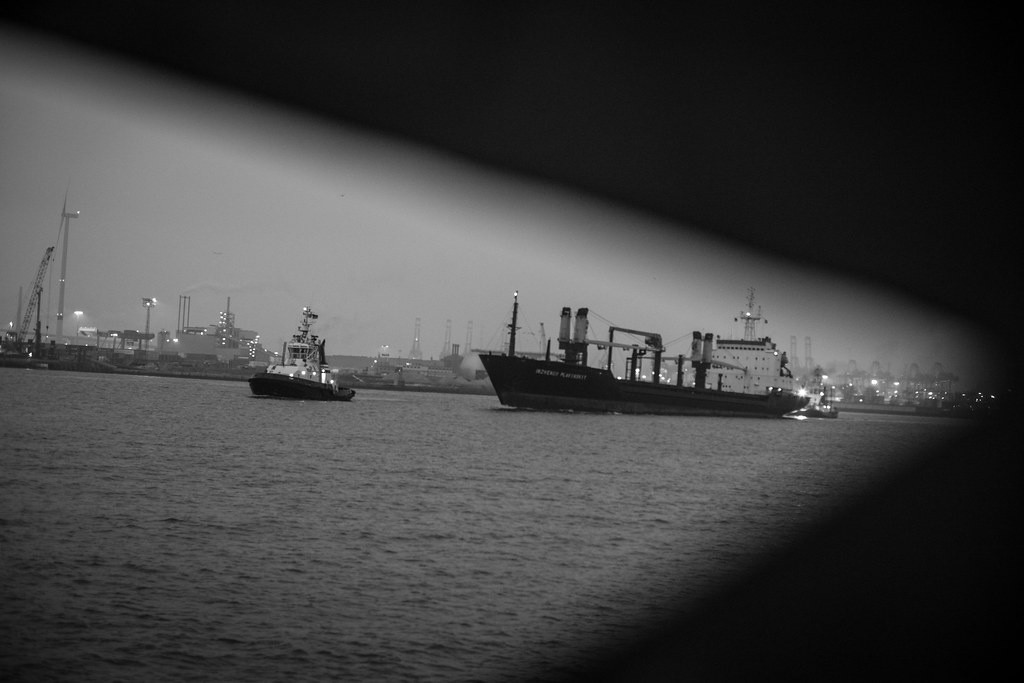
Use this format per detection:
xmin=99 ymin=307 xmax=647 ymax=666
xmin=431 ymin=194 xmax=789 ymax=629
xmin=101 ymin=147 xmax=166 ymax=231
xmin=18 ymin=246 xmax=57 ymax=343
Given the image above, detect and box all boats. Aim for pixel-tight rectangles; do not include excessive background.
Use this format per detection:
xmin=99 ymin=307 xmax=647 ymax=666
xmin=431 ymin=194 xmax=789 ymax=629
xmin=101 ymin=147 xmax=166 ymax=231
xmin=0 ymin=329 xmax=52 ymax=369
xmin=477 ymin=285 xmax=813 ymax=419
xmin=248 ymin=305 xmax=357 ymax=401
xmin=789 ymin=384 xmax=839 ymax=419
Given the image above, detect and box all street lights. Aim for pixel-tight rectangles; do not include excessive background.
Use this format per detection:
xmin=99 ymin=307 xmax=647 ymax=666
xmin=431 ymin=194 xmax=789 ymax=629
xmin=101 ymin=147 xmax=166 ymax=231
xmin=73 ymin=310 xmax=85 ymax=346
xmin=142 ymin=298 xmax=157 ymax=350
